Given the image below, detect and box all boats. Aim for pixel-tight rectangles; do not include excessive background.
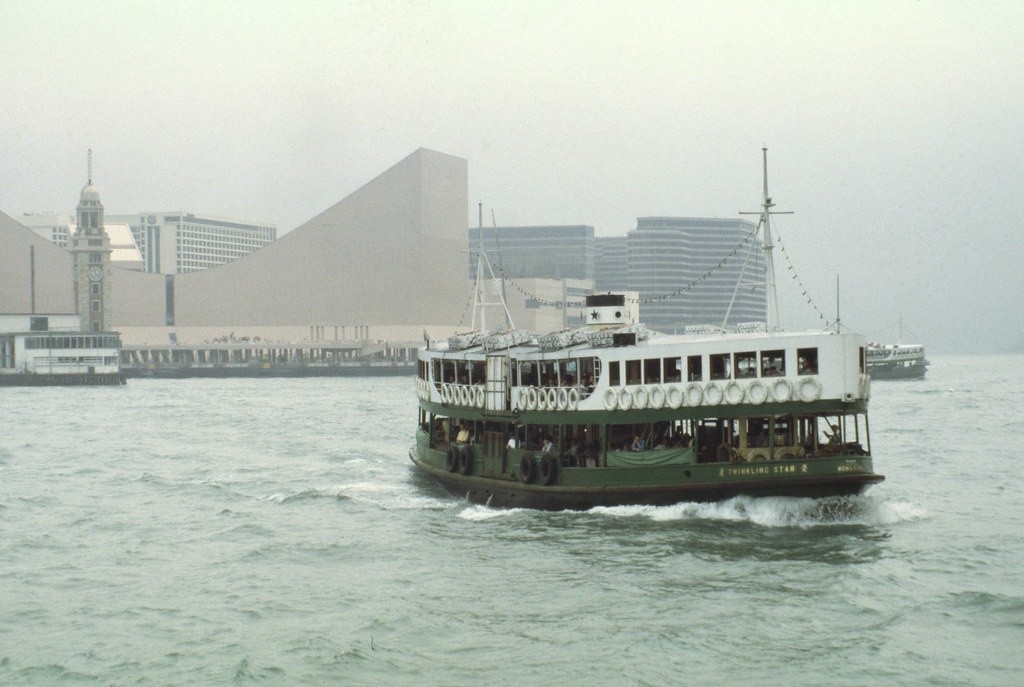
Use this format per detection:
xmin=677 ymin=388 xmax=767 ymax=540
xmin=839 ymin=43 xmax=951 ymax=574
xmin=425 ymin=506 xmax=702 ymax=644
xmin=408 ymin=141 xmax=885 ymax=511
xmin=822 ymin=274 xmax=930 ymax=381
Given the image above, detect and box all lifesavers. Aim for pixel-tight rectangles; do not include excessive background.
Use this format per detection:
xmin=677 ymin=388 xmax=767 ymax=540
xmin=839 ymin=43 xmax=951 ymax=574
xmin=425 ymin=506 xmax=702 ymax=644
xmin=445 ymin=446 xmax=458 ymax=473
xmin=519 ymin=454 xmax=537 ymax=485
xmin=770 ymin=378 xmax=794 ymax=403
xmin=747 ymin=447 xmax=770 ymax=461
xmin=458 ymin=444 xmax=474 ymax=476
xmin=859 ymin=374 xmax=871 ymax=402
xmin=744 ymin=379 xmax=768 ymax=406
xmin=684 ymin=382 xmax=704 ymax=407
xmin=414 ymin=377 xmax=431 ymax=402
xmin=537 ymin=454 xmax=558 ymax=486
xmin=648 ymin=385 xmax=666 ymax=410
xmin=602 ymin=387 xmax=619 ymax=412
xmin=633 ymin=386 xmax=649 ymax=409
xmin=702 ymin=381 xmax=724 ymax=407
xmin=776 ymin=446 xmax=797 ymax=460
xmin=516 ymin=386 xmax=580 ymax=411
xmin=795 ymin=376 xmax=822 ymax=403
xmin=723 ymin=381 xmax=745 ymax=405
xmin=617 ymin=386 xmax=631 ymax=411
xmin=666 ymin=384 xmax=684 ymax=409
xmin=439 ymin=383 xmax=485 ymax=408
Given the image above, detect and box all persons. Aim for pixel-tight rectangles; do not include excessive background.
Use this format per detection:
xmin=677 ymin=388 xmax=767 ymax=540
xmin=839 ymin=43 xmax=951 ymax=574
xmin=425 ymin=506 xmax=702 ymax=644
xmin=653 ymin=437 xmax=690 ymax=450
xmin=456 ymin=423 xmax=470 ymax=445
xmin=564 ymin=438 xmax=579 ymax=457
xmin=581 ymin=376 xmax=587 ymax=386
xmin=542 ymin=437 xmax=555 ymax=452
xmin=632 ymin=435 xmax=645 ymax=451
xmin=506 ymin=432 xmax=521 ymax=449
xmin=799 ymin=356 xmax=816 ymax=375
xmin=545 ymin=376 xmax=568 ymax=387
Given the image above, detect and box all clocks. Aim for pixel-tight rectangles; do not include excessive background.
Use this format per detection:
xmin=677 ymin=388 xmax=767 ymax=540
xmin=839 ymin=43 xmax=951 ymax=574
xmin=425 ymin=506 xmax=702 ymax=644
xmin=89 ymin=266 xmax=102 ymax=281
